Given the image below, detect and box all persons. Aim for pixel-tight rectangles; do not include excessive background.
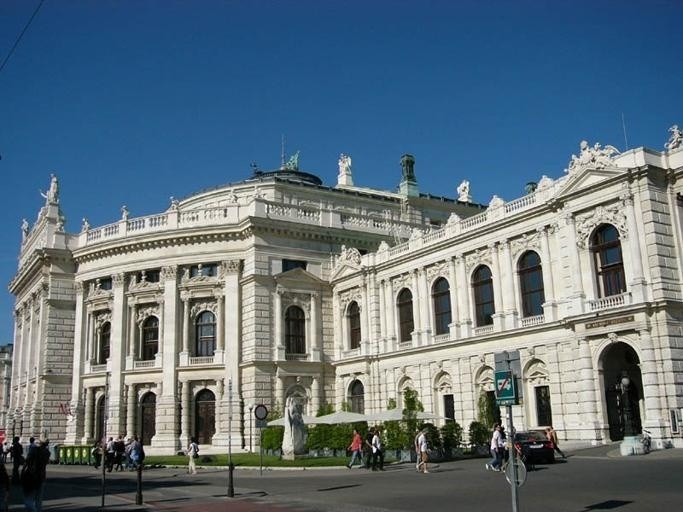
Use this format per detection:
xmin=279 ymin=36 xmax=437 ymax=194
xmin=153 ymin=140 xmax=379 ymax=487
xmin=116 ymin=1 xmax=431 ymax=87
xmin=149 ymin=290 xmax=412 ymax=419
xmin=0 ymin=428 xmax=53 ymax=512
xmin=17 ymin=168 xmax=180 ymax=246
xmin=186 ymin=436 xmax=199 ymax=475
xmin=91 ymin=435 xmax=145 ymax=473
xmin=335 ymin=151 xmax=355 ymax=187
xmin=347 ymin=424 xmax=568 ymax=471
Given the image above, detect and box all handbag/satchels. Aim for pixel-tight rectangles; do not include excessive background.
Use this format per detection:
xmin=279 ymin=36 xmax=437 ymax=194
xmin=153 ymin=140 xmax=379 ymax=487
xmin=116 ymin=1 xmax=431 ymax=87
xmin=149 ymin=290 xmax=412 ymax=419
xmin=193 ymin=453 xmax=199 ymax=459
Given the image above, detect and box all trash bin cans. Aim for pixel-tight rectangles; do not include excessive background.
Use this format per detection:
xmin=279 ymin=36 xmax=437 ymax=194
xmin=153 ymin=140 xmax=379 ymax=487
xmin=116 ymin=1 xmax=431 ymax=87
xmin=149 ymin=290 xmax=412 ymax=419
xmin=49 ymin=444 xmax=102 ymax=466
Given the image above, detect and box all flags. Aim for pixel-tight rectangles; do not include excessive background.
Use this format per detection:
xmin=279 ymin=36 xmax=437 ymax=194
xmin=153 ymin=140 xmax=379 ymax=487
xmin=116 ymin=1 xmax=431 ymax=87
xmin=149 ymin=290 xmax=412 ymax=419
xmin=60 ymin=404 xmax=73 ymax=417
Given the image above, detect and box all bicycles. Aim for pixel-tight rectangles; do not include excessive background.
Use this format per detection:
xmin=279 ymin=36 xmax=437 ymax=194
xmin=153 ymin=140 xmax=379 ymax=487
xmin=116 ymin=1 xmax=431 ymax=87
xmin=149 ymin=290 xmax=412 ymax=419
xmin=642 ymin=429 xmax=651 ymax=453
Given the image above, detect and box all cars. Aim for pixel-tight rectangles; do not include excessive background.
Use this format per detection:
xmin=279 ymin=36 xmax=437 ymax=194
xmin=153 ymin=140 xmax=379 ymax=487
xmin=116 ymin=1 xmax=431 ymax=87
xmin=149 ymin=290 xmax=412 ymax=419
xmin=503 ymin=430 xmax=554 ymax=465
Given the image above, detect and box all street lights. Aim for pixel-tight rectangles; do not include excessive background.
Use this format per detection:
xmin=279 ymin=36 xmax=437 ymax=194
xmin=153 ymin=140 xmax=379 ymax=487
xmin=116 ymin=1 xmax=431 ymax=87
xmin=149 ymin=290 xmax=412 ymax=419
xmin=619 ymin=369 xmax=644 ymax=457
xmin=248 ymin=403 xmax=252 ymax=455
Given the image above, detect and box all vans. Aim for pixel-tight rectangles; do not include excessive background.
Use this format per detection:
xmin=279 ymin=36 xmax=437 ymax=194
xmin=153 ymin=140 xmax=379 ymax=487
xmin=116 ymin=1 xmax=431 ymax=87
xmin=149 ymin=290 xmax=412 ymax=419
xmin=527 ymin=426 xmax=557 ymax=449
